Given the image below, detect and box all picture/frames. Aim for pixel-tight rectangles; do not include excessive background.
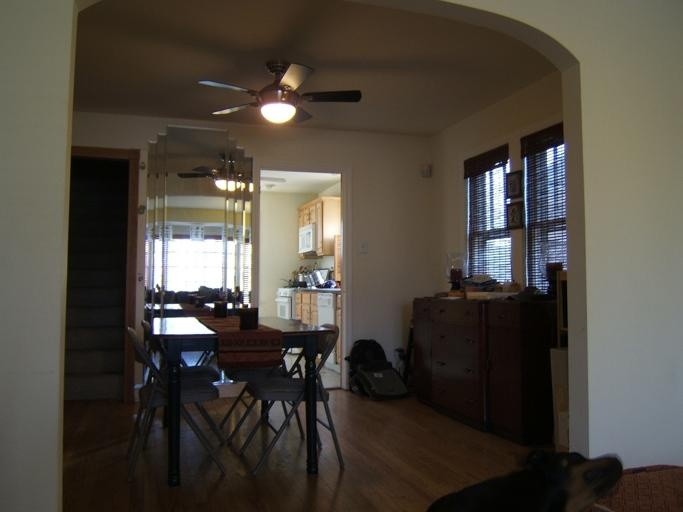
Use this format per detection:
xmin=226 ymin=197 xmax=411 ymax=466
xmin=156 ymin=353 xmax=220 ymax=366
xmin=506 ymin=201 xmax=525 ymax=230
xmin=506 ymin=170 xmax=523 ymax=198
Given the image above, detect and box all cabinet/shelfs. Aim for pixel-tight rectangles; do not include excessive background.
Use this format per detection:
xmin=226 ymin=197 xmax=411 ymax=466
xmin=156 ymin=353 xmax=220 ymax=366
xmin=335 ymin=291 xmax=342 ymax=365
xmin=298 ymin=196 xmax=341 ymax=259
xmin=302 ymin=291 xmax=317 ymax=325
xmin=414 ymin=297 xmax=556 ymax=447
xmin=295 ymin=292 xmax=301 ymax=320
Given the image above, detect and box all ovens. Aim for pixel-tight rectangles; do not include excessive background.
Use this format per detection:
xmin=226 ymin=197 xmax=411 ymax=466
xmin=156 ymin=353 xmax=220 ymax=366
xmin=275 ymin=297 xmax=291 ymax=320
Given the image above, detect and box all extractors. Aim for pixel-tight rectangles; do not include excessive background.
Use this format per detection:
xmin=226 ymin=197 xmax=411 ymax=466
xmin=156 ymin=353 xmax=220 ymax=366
xmin=299 ymin=224 xmax=315 ymax=254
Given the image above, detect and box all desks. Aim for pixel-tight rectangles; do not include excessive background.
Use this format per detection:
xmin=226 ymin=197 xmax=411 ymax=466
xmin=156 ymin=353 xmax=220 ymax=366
xmin=146 ymin=302 xmax=245 ymax=316
xmin=153 ymin=315 xmax=336 ymax=487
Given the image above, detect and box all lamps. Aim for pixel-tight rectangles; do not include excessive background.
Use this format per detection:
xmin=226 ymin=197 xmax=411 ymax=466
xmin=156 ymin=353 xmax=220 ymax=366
xmin=213 ymin=176 xmax=254 ymax=193
xmin=257 ymin=89 xmax=303 ymax=124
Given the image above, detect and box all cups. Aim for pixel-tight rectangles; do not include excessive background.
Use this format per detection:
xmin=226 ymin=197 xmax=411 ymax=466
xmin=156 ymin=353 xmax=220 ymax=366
xmin=239 ymin=308 xmax=258 ymax=330
xmin=213 ymin=300 xmax=227 ymax=318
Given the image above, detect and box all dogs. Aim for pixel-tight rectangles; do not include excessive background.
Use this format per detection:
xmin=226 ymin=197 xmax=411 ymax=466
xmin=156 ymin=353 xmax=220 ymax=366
xmin=426 ymin=449 xmax=623 ymax=512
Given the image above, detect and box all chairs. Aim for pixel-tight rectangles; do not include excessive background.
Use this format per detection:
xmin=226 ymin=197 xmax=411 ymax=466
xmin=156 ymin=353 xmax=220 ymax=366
xmin=228 ymin=323 xmax=343 ymax=476
xmin=141 ymin=319 xmax=225 ymax=450
xmin=219 ymin=318 xmax=305 ymax=443
xmin=126 ymin=327 xmax=229 ymax=482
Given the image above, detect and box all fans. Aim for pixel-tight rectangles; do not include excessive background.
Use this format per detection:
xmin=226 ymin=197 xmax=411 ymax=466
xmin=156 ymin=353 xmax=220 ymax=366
xmin=197 ymin=63 xmax=362 ymax=123
xmin=176 ymin=152 xmax=250 ymax=178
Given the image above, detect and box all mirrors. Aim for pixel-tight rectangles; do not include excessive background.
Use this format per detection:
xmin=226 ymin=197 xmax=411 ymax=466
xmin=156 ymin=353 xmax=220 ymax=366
xmin=146 ymin=126 xmax=259 ymax=384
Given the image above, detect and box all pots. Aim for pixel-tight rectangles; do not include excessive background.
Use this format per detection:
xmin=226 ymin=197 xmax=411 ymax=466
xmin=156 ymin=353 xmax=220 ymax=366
xmin=304 ymin=270 xmax=325 ymax=287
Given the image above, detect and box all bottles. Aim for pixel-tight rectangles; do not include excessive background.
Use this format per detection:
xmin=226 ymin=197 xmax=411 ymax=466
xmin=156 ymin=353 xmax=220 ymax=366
xmin=329 ymin=267 xmax=334 ymax=280
xmin=295 ymin=272 xmax=304 ymax=282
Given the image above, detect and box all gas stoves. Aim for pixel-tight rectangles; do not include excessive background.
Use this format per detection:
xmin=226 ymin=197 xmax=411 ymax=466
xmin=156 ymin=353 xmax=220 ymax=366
xmin=276 ymin=287 xmax=301 ymax=297
